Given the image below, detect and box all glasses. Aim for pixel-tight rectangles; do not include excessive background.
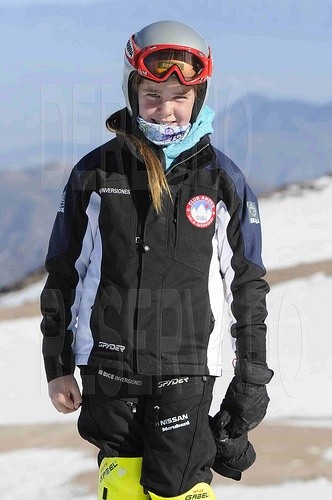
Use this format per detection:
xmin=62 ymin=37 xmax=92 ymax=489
xmin=125 ymin=32 xmax=213 ymax=84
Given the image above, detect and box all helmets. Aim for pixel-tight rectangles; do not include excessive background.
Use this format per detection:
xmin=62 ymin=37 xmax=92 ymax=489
xmin=121 ymin=20 xmax=211 ymax=123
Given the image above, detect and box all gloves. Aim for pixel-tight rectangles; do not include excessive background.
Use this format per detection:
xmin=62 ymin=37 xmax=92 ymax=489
xmin=220 ymin=359 xmax=274 ymax=439
xmin=208 ymin=412 xmax=256 ymax=480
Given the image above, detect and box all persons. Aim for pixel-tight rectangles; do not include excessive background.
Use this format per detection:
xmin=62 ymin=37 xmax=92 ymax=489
xmin=41 ymin=19 xmax=275 ymax=500
